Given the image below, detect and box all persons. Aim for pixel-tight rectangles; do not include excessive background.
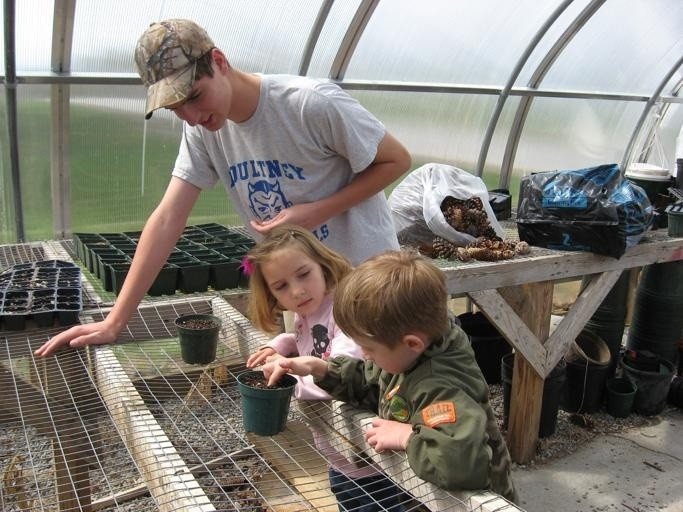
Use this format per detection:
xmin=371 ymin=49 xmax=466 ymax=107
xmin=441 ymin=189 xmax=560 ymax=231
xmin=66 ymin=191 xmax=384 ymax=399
xmin=244 ymin=223 xmax=432 ymax=511
xmin=32 ymin=17 xmax=413 ymax=358
xmin=261 ymin=247 xmax=520 ymax=511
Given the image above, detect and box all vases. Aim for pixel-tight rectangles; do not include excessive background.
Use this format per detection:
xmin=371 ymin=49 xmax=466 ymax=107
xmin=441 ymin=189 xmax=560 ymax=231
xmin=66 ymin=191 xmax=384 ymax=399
xmin=625 ymin=158 xmax=682 ymax=237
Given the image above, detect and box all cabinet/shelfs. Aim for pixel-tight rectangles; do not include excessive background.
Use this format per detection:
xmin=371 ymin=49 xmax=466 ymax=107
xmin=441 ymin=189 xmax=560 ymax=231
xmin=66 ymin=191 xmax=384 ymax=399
xmin=0 ymin=204 xmax=683 ymax=512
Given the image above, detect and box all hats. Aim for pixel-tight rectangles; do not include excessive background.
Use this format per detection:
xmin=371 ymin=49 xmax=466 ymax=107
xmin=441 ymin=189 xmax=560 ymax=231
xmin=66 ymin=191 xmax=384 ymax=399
xmin=133 ymin=18 xmax=215 ymax=119
